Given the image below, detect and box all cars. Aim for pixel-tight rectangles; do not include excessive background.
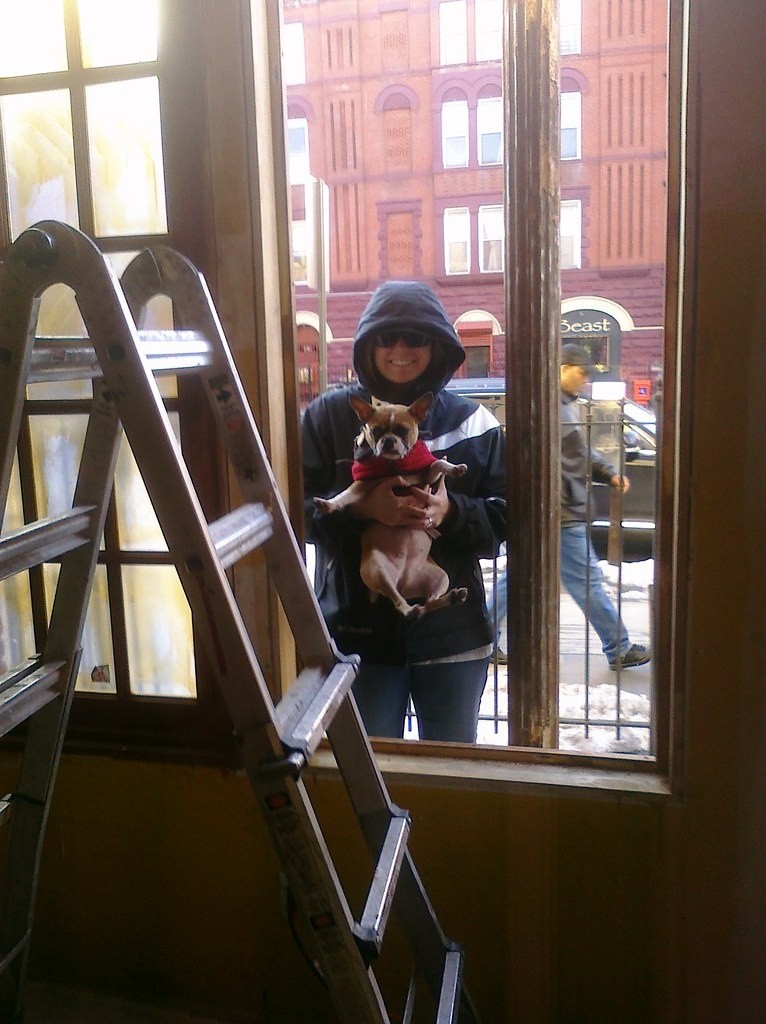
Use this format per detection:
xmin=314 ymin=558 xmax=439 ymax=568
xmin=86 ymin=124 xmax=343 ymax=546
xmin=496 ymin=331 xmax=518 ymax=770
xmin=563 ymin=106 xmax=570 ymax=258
xmin=442 ymin=378 xmax=659 ymax=523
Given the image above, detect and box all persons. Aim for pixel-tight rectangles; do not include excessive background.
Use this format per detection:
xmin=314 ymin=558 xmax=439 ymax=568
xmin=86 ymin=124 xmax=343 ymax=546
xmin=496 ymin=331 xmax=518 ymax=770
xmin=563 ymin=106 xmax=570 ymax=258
xmin=300 ymin=281 xmax=505 ymax=742
xmin=487 ymin=344 xmax=652 ymax=670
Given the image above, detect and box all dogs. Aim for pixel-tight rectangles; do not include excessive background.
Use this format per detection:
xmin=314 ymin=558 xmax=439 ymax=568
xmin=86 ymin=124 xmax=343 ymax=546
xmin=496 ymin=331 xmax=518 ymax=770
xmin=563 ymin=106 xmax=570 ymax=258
xmin=311 ymin=392 xmax=468 ymax=622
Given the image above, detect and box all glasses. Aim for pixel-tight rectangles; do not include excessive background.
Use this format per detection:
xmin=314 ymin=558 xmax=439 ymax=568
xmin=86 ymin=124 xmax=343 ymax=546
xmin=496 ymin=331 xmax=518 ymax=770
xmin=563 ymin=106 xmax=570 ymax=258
xmin=373 ymin=331 xmax=428 ymax=347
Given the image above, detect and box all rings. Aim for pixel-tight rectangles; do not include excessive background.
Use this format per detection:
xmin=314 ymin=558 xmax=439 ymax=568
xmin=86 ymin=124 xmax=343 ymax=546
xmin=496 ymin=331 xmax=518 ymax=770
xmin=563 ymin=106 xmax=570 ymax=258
xmin=429 ymin=518 xmax=432 ymax=523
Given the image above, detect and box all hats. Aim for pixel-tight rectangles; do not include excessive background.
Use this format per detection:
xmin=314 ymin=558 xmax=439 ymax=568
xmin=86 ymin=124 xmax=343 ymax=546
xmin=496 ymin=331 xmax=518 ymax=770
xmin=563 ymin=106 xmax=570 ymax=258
xmin=560 ymin=344 xmax=600 ymax=376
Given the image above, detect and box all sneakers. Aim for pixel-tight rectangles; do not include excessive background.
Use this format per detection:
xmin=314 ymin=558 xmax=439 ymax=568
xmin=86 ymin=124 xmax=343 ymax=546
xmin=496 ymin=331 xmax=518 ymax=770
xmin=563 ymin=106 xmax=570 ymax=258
xmin=489 ymin=647 xmax=508 ymax=664
xmin=609 ymin=643 xmax=652 ymax=669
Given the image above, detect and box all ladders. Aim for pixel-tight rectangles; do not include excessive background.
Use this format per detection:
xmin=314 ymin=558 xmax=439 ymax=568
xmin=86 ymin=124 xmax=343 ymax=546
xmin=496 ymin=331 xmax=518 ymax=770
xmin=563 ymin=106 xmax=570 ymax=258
xmin=0 ymin=219 xmax=486 ymax=1023
